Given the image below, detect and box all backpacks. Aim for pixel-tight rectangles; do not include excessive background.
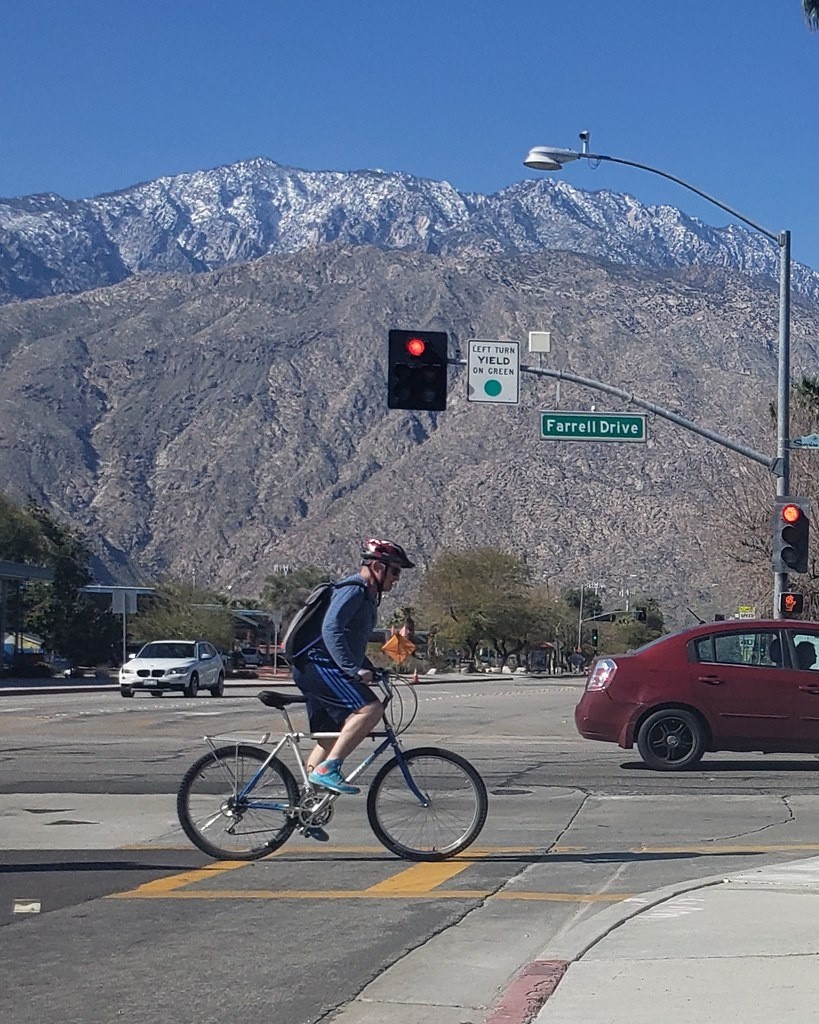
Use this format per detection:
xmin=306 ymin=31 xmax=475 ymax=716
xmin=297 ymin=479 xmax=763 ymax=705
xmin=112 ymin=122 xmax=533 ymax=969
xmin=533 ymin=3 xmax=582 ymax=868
xmin=281 ymin=580 xmax=367 ymax=663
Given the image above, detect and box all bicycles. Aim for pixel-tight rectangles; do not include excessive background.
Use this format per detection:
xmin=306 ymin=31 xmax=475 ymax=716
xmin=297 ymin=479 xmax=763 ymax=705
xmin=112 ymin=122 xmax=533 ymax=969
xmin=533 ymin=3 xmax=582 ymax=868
xmin=176 ymin=665 xmax=490 ymax=863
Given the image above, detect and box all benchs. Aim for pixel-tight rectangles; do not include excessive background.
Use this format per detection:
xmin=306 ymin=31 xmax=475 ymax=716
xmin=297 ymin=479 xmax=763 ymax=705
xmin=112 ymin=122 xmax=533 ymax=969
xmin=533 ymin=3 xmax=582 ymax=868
xmin=70 ymin=649 xmax=109 ymax=679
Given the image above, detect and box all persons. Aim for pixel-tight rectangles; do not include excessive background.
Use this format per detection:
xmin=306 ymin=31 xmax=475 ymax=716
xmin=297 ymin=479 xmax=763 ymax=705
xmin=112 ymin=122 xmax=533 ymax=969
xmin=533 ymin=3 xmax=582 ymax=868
xmin=795 ymin=641 xmax=817 ymax=668
xmin=284 ymin=539 xmax=416 ymax=841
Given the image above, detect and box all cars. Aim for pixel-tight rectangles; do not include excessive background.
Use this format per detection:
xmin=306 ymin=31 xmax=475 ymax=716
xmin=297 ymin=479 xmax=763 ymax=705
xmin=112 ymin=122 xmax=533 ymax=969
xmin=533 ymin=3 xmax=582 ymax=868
xmin=118 ymin=639 xmax=226 ymax=699
xmin=218 ymin=651 xmax=235 ymax=675
xmin=242 ymin=648 xmax=263 ymax=667
xmin=229 ymin=649 xmax=246 ymax=667
xmin=270 ymin=651 xmax=288 ymax=666
xmin=573 ymin=616 xmax=819 ymax=771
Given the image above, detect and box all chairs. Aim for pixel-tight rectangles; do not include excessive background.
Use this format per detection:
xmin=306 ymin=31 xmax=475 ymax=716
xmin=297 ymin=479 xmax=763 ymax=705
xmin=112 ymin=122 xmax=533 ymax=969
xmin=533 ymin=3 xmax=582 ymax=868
xmin=770 ymin=639 xmax=782 ymax=667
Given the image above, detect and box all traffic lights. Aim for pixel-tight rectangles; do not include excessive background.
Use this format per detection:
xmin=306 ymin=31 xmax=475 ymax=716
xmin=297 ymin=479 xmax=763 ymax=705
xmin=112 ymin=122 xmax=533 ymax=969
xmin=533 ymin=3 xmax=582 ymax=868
xmin=772 ymin=496 xmax=810 ymax=572
xmin=591 ymin=628 xmax=598 ymax=646
xmin=387 ymin=328 xmax=448 ymax=411
xmin=780 ymin=591 xmax=804 ymax=616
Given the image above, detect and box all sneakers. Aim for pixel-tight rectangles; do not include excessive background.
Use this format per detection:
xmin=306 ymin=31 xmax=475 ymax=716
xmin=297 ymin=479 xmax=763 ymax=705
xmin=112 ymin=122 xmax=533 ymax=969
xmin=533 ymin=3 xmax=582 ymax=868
xmin=308 ymin=758 xmax=361 ymax=794
xmin=299 ymin=820 xmax=329 ymax=842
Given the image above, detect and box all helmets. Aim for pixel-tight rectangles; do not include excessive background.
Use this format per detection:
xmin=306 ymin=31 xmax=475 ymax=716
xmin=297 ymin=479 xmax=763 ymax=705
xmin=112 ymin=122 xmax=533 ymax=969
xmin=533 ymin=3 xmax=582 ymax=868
xmin=360 ymin=538 xmax=415 ymax=568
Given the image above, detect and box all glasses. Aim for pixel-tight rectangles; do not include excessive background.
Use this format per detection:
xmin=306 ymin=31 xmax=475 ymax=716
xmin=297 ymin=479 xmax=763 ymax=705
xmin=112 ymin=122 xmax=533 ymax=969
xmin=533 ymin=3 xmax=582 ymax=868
xmin=381 ymin=561 xmax=401 ymax=576
